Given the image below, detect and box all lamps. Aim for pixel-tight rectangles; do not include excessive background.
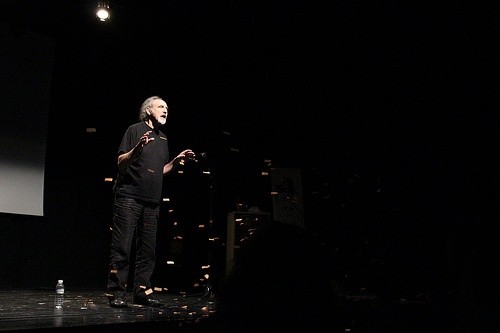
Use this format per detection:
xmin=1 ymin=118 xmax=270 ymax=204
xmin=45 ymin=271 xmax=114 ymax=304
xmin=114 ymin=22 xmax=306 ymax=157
xmin=95 ymin=0 xmax=112 ymax=25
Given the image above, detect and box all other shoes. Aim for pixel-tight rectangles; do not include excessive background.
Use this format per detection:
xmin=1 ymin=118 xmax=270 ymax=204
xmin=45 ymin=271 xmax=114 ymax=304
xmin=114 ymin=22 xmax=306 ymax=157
xmin=110 ymin=298 xmax=127 ymax=307
xmin=135 ymin=297 xmax=165 ymax=307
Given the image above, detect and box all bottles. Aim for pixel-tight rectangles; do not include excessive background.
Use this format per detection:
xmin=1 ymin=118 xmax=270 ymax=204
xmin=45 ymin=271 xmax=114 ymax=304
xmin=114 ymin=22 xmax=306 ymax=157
xmin=55 ymin=280 xmax=64 ymax=309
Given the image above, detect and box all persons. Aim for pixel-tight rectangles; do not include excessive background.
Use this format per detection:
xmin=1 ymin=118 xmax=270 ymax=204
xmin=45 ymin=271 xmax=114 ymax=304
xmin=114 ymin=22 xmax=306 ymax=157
xmin=108 ymin=95 xmax=195 ymax=307
